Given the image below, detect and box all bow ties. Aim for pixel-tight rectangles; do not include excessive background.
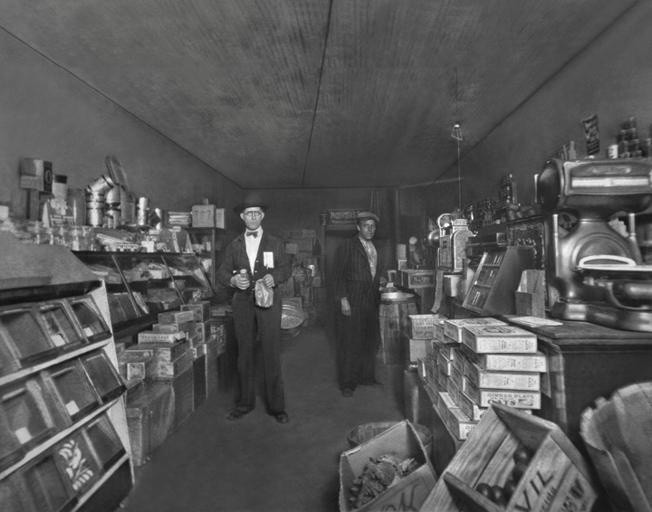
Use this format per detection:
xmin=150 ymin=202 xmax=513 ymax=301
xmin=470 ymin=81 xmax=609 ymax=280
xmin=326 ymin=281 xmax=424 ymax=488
xmin=246 ymin=231 xmax=258 ymax=237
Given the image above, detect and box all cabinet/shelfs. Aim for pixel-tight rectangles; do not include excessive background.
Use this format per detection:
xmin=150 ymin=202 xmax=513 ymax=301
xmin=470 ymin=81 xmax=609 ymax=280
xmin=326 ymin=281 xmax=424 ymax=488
xmin=0 ymin=227 xmax=240 ymax=512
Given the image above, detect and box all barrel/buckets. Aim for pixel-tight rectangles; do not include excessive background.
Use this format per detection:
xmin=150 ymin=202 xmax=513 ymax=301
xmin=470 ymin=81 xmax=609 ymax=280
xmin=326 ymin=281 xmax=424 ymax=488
xmin=375 ymin=291 xmax=421 ymax=388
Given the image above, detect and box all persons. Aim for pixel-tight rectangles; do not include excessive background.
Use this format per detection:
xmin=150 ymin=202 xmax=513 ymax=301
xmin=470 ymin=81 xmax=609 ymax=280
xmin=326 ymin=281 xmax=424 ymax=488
xmin=215 ymin=194 xmax=292 ymax=424
xmin=332 ymin=212 xmax=388 ymax=397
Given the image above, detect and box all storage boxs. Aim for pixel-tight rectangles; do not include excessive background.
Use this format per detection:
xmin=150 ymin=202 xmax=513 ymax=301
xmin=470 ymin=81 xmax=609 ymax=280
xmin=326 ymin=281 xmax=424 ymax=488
xmin=338 ymin=418 xmax=439 ymax=511
xmin=217 ymin=207 xmax=227 ymax=230
xmin=393 ymin=258 xmax=448 ymax=361
xmin=420 ymin=400 xmax=603 ymax=512
xmin=191 ymin=204 xmax=217 ymax=228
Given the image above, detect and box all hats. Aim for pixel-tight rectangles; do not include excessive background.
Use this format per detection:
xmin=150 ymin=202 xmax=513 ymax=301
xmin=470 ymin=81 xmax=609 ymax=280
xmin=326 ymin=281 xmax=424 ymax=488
xmin=233 ymin=192 xmax=269 ymax=213
xmin=357 ymin=211 xmax=380 ymax=223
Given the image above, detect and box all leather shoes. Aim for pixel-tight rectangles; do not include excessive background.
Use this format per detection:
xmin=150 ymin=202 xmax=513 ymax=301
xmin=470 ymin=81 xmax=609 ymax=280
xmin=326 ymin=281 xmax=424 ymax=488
xmin=343 ymin=387 xmax=355 ymax=398
xmin=359 ymin=380 xmax=383 ymax=388
xmin=268 ymin=408 xmax=287 ymax=423
xmin=227 ymin=407 xmax=255 ymax=420
